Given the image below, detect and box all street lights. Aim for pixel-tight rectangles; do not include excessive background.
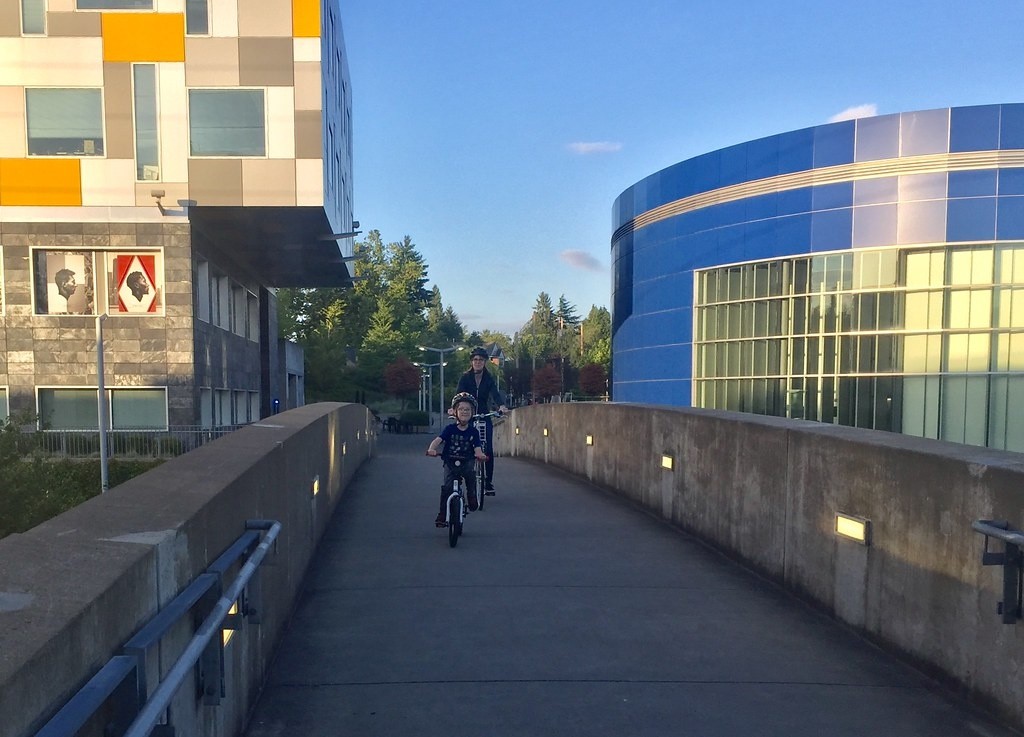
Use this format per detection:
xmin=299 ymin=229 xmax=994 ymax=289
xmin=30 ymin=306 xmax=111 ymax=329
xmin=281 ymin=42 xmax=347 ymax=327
xmin=410 ymin=344 xmax=465 ymax=436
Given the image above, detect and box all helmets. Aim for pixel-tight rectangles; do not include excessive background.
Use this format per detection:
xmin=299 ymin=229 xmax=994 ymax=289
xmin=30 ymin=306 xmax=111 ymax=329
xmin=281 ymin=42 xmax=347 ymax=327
xmin=451 ymin=391 xmax=477 ymax=415
xmin=469 ymin=346 xmax=489 ymax=359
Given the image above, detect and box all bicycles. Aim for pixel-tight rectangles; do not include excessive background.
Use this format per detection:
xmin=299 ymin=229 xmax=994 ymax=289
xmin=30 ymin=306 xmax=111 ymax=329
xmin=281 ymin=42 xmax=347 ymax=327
xmin=426 ymin=450 xmax=490 ymax=548
xmin=445 ymin=406 xmax=511 ymax=511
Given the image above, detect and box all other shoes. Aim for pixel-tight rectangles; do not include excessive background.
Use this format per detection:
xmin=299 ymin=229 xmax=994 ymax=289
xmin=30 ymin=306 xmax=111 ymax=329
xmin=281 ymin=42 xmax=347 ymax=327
xmin=485 ymin=481 xmax=496 ymax=493
xmin=466 ymin=492 xmax=480 ymax=512
xmin=435 ymin=514 xmax=447 ymax=524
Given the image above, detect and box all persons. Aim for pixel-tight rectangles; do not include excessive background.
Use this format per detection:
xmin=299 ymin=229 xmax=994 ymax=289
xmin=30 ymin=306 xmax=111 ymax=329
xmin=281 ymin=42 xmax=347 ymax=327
xmin=47 ymin=269 xmax=78 ymax=313
xmin=427 ymin=392 xmax=488 ymax=526
xmin=124 ymin=271 xmax=150 ymax=313
xmin=447 ymin=346 xmax=509 ymax=493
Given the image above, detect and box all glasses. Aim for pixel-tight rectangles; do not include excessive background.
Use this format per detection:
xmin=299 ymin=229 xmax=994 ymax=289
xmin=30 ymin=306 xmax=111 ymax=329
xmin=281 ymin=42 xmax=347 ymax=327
xmin=456 ymin=407 xmax=473 ymax=414
xmin=472 ymin=357 xmax=485 ymax=362
